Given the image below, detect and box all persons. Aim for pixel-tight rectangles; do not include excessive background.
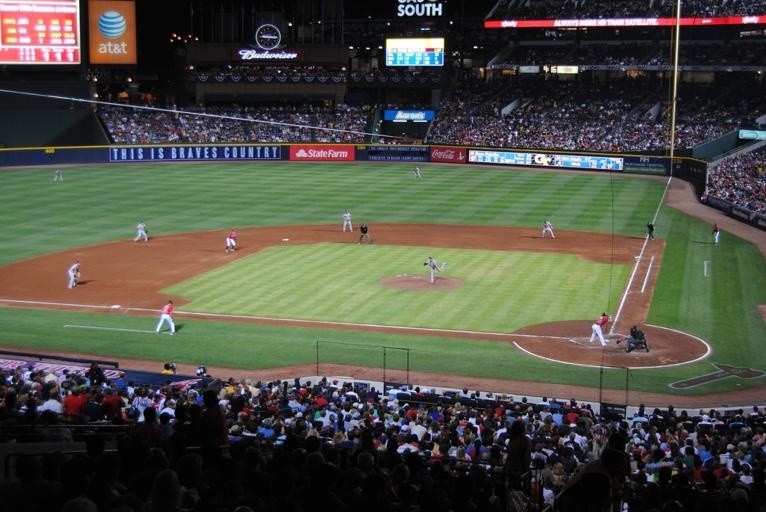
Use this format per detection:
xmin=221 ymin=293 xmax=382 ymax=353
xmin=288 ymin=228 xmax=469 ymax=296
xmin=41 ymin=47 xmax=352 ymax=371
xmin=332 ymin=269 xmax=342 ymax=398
xmin=414 ymin=167 xmax=423 ymax=181
xmin=424 ymin=256 xmax=446 ymax=283
xmin=133 ymin=221 xmax=148 ymax=244
xmin=542 ymin=221 xmax=555 ymax=239
xmin=1 ymin=360 xmax=765 ymax=512
xmin=590 ymin=311 xmax=650 ymax=353
xmin=67 ymin=261 xmax=82 ymax=289
xmin=711 ymin=223 xmax=721 ymax=243
xmin=357 ymin=224 xmax=371 ymax=243
xmin=708 ymin=147 xmax=765 ymax=214
xmin=225 ymin=229 xmax=237 ymax=251
xmin=156 ymin=300 xmax=176 ymax=336
xmin=88 ymin=1 xmax=766 ymax=153
xmin=342 ymin=208 xmax=353 ymax=231
xmin=54 ymin=169 xmax=63 ymax=183
xmin=647 ymin=223 xmax=655 ymax=239
